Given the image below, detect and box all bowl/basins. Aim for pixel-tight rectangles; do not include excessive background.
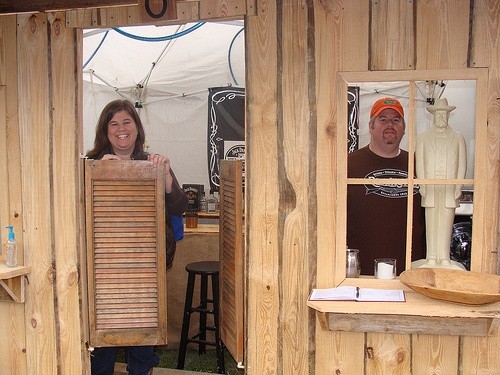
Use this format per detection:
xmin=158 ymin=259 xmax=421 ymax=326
xmin=399 ymin=267 xmax=500 ymax=307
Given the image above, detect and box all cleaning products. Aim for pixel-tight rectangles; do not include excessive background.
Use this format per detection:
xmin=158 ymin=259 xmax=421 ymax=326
xmin=4 ymin=225 xmax=18 ymax=268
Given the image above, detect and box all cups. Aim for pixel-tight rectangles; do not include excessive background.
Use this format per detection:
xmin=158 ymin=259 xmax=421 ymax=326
xmin=184 ymin=208 xmax=198 ymax=228
xmin=373 ymin=257 xmax=397 ymax=280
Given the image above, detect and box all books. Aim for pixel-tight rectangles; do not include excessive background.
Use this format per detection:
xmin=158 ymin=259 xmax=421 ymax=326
xmin=309 ymin=286 xmax=406 ymax=302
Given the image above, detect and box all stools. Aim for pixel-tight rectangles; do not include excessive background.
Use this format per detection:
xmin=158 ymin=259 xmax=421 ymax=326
xmin=175 ymin=261 xmax=227 ymax=374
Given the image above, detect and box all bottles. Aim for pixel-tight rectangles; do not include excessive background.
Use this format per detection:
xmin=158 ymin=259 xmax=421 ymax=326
xmin=205 ymin=189 xmax=218 ymax=214
xmin=345 ymin=248 xmax=361 ymax=278
xmin=200 ymin=191 xmax=207 ymax=212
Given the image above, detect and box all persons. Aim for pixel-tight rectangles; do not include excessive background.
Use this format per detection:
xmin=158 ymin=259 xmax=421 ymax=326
xmin=84 ymin=100 xmax=189 ymax=375
xmin=347 ymin=98 xmax=427 ymax=275
xmin=416 ymin=100 xmax=467 ymax=265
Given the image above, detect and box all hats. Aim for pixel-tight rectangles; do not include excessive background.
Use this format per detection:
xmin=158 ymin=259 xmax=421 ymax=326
xmin=370 ymin=97 xmax=404 ymax=122
